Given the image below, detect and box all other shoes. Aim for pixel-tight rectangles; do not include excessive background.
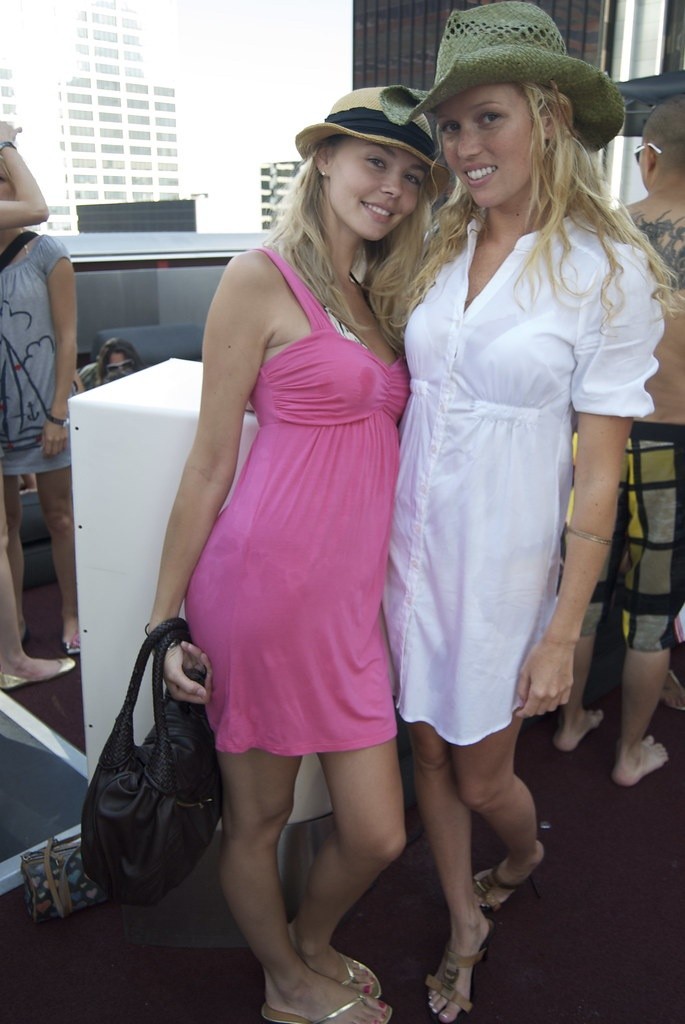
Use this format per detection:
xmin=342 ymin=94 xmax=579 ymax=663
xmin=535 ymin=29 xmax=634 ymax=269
xmin=0 ymin=657 xmax=76 ymax=691
xmin=662 ymin=669 xmax=685 ymax=711
xmin=63 ymin=631 xmax=79 ymax=655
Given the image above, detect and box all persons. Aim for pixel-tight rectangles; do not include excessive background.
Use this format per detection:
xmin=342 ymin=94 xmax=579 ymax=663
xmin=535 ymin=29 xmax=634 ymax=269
xmin=0 ymin=122 xmax=144 ymax=691
xmin=380 ymin=0 xmax=662 ymax=1023
xmin=144 ymin=88 xmax=408 ymax=1023
xmin=552 ymin=101 xmax=684 ymax=787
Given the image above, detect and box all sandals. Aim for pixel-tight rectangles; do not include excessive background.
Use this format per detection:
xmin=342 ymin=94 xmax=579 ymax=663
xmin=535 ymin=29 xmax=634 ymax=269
xmin=424 ymin=917 xmax=495 ymax=1023
xmin=471 ymin=864 xmax=520 ymax=912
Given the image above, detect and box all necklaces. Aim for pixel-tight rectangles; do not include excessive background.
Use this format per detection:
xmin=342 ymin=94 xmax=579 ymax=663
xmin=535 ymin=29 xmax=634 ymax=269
xmin=20 ymin=227 xmax=29 ymax=256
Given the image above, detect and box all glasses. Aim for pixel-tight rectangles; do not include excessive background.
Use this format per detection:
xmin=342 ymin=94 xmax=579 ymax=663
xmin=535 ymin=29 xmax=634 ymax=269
xmin=634 ymin=142 xmax=662 ymax=163
xmin=105 ymin=359 xmax=135 ymax=373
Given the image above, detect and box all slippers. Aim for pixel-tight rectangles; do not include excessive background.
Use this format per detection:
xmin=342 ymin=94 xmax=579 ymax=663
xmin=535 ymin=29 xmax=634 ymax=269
xmin=262 ymin=952 xmax=393 ymax=1024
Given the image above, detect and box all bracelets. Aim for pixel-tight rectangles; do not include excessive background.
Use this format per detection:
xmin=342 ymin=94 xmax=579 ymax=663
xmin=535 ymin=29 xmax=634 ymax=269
xmin=0 ymin=141 xmax=18 ymax=151
xmin=566 ymin=526 xmax=613 ymax=546
xmin=145 ymin=623 xmax=176 ymax=646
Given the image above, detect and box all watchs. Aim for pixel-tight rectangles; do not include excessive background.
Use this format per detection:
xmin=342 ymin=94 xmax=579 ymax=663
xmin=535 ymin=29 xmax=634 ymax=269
xmin=47 ymin=415 xmax=69 ymax=426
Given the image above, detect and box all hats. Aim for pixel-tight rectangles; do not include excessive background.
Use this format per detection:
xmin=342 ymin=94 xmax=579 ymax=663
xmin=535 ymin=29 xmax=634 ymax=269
xmin=295 ymin=86 xmax=452 ymax=195
xmin=380 ymin=1 xmax=625 ymax=153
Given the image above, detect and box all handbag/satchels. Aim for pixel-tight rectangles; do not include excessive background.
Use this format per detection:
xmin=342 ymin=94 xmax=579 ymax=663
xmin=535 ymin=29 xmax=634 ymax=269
xmin=22 ymin=836 xmax=113 ymax=923
xmin=79 ymin=616 xmax=224 ymax=906
